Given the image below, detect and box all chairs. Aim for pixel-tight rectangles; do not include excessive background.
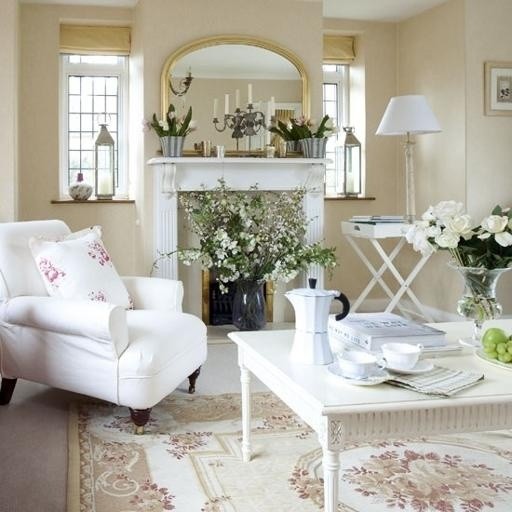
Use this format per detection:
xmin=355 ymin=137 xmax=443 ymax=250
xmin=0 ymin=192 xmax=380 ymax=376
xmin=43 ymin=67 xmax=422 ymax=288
xmin=0 ymin=216 xmax=207 ymax=435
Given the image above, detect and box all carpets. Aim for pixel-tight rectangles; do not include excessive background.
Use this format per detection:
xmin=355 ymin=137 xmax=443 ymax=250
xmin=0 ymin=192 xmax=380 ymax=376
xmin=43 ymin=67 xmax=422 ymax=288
xmin=62 ymin=389 xmax=512 ymax=512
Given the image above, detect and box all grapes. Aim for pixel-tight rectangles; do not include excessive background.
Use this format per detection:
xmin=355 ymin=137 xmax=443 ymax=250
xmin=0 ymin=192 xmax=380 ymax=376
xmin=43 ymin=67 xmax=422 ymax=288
xmin=484 ymin=336 xmax=512 ymax=362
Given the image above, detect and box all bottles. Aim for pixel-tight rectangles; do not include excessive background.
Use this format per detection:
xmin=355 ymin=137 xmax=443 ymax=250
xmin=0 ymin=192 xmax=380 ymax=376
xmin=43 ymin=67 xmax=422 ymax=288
xmin=278 ymin=137 xmax=286 ymax=158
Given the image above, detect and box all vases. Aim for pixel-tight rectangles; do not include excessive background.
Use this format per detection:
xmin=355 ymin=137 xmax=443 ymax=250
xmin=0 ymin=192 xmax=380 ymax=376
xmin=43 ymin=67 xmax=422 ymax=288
xmin=159 ymin=135 xmax=184 ymax=157
xmin=446 ymin=260 xmax=512 ymax=348
xmin=299 ymin=137 xmax=328 ymax=158
xmin=232 ymin=280 xmax=268 ymax=332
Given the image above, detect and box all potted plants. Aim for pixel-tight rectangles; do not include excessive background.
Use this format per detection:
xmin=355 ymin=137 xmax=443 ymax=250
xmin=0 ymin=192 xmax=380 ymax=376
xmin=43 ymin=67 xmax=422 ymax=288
xmin=286 ymin=140 xmax=305 ymax=154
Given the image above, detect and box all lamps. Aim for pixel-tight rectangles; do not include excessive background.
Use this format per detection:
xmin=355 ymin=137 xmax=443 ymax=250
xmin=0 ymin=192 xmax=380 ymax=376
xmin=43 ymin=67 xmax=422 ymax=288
xmin=375 ymin=94 xmax=444 ymax=222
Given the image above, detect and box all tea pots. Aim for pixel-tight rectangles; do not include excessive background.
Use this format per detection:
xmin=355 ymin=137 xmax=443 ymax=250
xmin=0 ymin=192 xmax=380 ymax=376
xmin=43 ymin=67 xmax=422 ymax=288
xmin=279 ymin=278 xmax=348 ymax=332
xmin=288 ymin=332 xmax=335 ymax=366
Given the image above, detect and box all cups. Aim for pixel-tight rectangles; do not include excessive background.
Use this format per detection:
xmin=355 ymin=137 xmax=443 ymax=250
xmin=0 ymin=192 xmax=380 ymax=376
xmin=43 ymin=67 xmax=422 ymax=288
xmin=336 ymin=352 xmax=387 ymax=378
xmin=265 ymin=144 xmax=275 ymax=158
xmin=215 ymin=146 xmax=224 ymax=158
xmin=201 ymin=141 xmax=212 ymax=157
xmin=381 ymin=342 xmax=424 ymax=371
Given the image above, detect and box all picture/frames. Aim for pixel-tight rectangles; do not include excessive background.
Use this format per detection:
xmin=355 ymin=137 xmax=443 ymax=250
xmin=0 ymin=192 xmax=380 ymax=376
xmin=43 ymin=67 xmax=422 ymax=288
xmin=485 ymin=60 xmax=512 ymax=119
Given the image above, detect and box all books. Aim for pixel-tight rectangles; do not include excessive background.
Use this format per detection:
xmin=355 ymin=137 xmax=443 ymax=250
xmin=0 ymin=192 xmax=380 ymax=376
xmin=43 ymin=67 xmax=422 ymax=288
xmin=348 ymin=215 xmax=406 ymax=223
xmin=327 ymin=311 xmax=446 ymax=350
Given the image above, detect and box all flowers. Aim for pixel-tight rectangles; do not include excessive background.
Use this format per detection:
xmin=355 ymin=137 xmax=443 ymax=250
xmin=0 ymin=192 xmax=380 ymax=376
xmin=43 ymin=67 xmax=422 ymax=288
xmin=268 ymin=116 xmax=294 ymax=141
xmin=147 ymin=101 xmax=197 ymax=136
xmin=140 ymin=168 xmax=341 ymax=285
xmin=406 ymin=197 xmax=512 ymax=322
xmin=288 ymin=114 xmax=339 ymax=138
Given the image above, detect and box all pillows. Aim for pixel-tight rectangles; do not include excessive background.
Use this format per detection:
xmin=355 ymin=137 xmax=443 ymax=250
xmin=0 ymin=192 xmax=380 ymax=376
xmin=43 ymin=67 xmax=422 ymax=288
xmin=28 ymin=224 xmax=135 ymax=311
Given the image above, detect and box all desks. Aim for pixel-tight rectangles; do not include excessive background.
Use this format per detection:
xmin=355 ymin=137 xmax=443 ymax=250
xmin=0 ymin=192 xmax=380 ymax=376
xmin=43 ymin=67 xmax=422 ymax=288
xmin=338 ymin=218 xmax=444 ymax=324
xmin=224 ymin=318 xmax=512 ymax=511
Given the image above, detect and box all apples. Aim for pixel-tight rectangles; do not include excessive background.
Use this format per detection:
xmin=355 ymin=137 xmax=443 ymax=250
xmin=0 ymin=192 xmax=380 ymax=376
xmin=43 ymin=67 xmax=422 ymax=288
xmin=483 ymin=328 xmax=507 ymax=344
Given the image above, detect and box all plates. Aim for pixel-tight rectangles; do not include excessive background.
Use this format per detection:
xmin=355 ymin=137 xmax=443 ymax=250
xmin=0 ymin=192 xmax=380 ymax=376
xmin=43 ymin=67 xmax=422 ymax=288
xmin=474 ymin=348 xmax=511 ymax=371
xmin=375 ymin=353 xmax=434 ymax=374
xmin=327 ymin=362 xmax=390 ymax=386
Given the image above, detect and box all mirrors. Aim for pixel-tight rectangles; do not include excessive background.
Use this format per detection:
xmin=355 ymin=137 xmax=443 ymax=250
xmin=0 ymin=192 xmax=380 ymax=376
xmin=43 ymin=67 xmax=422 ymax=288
xmin=157 ymin=36 xmax=310 ymax=157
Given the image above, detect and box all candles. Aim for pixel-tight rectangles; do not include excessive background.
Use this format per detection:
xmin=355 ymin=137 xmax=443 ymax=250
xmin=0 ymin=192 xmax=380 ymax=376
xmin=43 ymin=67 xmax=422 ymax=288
xmin=211 ymin=83 xmax=277 ymax=120
xmin=98 ymin=180 xmax=110 ymax=195
xmin=341 ymin=171 xmax=354 ymax=193
